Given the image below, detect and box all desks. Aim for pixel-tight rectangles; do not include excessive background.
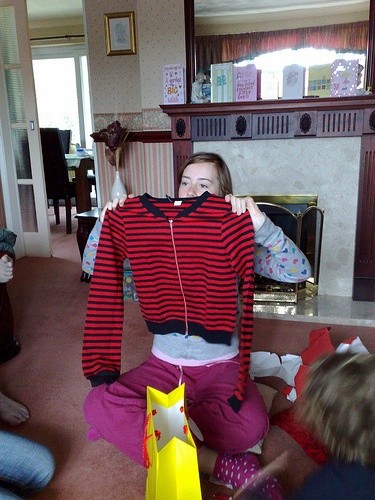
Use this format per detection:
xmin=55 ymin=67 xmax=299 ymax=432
xmin=74 ymin=209 xmax=112 ymax=284
xmin=57 ymin=152 xmax=94 ymax=212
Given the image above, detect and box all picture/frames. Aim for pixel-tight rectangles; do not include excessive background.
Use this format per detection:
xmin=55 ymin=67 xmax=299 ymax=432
xmin=102 ymin=11 xmax=135 ymax=57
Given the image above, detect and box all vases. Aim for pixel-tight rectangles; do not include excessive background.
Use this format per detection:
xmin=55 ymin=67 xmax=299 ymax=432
xmin=110 ymin=171 xmax=128 ymax=203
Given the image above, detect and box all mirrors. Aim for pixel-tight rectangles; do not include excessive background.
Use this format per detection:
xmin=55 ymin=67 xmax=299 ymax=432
xmin=185 ymin=0 xmax=374 ymax=100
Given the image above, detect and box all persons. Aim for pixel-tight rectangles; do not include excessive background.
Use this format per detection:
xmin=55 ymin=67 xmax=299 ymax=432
xmin=81 ymin=153 xmax=312 ymax=500
xmin=0 ymin=254 xmax=57 ymax=500
xmin=283 ymin=353 xmax=375 ymax=500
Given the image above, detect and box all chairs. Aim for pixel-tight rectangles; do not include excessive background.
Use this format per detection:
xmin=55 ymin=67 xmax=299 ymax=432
xmin=59 ymin=128 xmax=71 ymax=154
xmin=71 ymin=175 xmax=97 ymax=188
xmin=21 ymin=127 xmax=79 ymax=234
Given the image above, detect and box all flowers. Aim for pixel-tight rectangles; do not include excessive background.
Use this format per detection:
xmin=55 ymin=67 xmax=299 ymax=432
xmin=90 ymin=120 xmax=131 ymax=151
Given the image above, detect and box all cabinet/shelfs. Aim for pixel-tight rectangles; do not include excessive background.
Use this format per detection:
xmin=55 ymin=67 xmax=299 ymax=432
xmin=157 ymin=95 xmax=375 ymax=303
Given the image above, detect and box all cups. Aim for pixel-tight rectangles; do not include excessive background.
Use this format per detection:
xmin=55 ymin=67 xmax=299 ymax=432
xmin=78 ymin=148 xmax=86 ymax=155
